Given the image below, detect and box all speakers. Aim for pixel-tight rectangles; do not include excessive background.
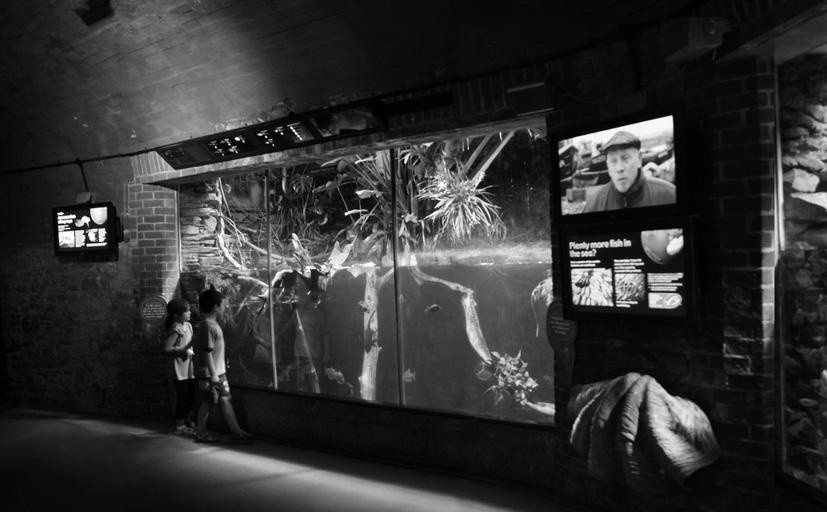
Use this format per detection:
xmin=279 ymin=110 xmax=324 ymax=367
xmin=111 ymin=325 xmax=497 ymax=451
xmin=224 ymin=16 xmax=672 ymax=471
xmin=657 ymin=16 xmax=724 ymax=64
xmin=505 ymin=80 xmax=556 ymax=118
xmin=74 ymin=0 xmax=113 ymax=26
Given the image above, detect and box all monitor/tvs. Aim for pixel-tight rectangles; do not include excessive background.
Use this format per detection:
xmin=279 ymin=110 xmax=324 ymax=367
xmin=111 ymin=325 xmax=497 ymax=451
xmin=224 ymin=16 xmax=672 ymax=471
xmin=559 ymin=213 xmax=699 ymax=328
xmin=51 ymin=201 xmax=123 ymax=264
xmin=551 ymin=102 xmax=682 ymax=225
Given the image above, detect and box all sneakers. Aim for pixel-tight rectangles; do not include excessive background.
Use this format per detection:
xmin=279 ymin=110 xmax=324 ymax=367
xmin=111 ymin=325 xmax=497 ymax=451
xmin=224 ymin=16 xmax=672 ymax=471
xmin=229 ymin=427 xmax=254 ymax=446
xmin=190 ymin=430 xmax=220 ymax=445
xmin=167 ymin=422 xmax=199 ymax=438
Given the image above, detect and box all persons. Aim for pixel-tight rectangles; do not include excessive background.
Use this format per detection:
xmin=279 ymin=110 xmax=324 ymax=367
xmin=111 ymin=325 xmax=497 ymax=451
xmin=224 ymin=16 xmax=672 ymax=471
xmin=581 ymin=129 xmax=675 ymax=215
xmin=194 ymin=289 xmax=256 ymax=445
xmin=162 ymin=294 xmax=198 ymax=439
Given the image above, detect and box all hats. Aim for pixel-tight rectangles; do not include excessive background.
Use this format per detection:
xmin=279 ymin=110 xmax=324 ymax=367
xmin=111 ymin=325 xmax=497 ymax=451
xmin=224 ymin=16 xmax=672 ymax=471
xmin=601 ymin=130 xmax=643 ymax=153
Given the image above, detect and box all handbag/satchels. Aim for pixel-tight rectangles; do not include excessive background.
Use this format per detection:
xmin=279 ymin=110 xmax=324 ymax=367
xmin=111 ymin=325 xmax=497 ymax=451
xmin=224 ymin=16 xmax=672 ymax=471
xmin=148 ymin=342 xmax=179 ymax=379
